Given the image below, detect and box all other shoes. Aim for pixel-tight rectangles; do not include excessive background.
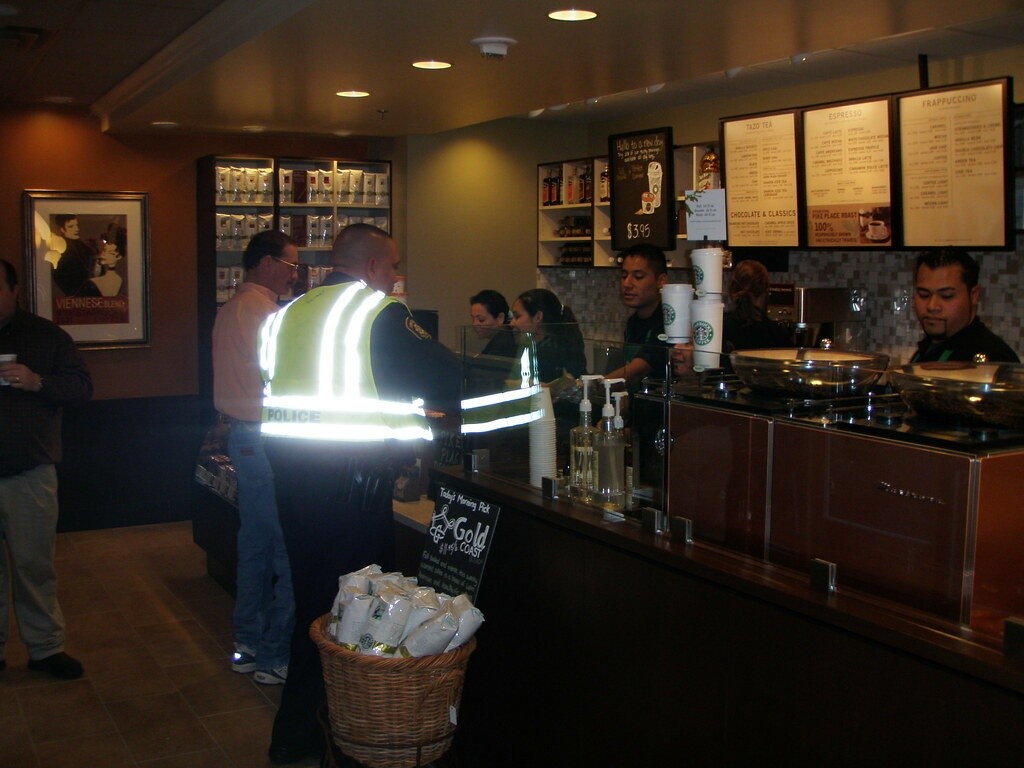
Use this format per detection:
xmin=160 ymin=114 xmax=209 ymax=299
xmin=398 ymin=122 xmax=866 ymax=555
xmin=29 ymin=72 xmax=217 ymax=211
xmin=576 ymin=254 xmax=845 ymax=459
xmin=29 ymin=651 xmax=81 ymax=679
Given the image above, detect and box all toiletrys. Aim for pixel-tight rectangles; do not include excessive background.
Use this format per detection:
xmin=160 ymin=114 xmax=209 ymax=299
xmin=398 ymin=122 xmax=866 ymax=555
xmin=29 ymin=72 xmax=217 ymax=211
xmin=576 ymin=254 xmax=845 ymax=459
xmin=569 ymin=374 xmax=605 ymax=503
xmin=591 ymin=377 xmax=627 ymax=512
xmin=610 ymin=391 xmax=634 ymax=512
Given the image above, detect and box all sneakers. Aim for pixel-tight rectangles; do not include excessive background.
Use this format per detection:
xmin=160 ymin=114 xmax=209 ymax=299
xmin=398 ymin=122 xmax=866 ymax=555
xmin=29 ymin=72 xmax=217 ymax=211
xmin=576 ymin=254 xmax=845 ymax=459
xmin=232 ymin=647 xmax=257 ymax=672
xmin=254 ymin=664 xmax=288 ymax=684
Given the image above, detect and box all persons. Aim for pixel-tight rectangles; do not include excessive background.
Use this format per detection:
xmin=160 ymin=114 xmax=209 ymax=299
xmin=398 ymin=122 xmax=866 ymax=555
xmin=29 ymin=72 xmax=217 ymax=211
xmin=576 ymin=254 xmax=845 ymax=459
xmin=668 ymin=324 xmax=699 ymax=376
xmin=903 ymin=246 xmax=1021 ymax=367
xmin=719 ymin=261 xmax=791 ymax=365
xmin=249 ymin=222 xmax=575 ymax=768
xmin=574 ymin=244 xmax=675 ymax=489
xmin=0 ymin=259 xmax=96 ymax=681
xmin=461 ymin=283 xmax=517 ymax=379
xmin=506 ymin=289 xmax=590 ymax=472
xmin=211 ymin=228 xmax=290 ymax=685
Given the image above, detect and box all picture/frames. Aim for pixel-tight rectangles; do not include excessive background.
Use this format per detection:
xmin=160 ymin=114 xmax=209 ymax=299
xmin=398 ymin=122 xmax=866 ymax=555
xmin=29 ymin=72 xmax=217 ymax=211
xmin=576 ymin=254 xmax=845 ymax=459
xmin=24 ymin=191 xmax=156 ymax=352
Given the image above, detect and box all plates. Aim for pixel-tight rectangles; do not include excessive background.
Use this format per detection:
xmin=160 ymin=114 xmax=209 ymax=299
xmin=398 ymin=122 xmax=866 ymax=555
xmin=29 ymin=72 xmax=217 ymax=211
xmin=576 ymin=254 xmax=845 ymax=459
xmin=866 ymin=231 xmax=889 ymax=240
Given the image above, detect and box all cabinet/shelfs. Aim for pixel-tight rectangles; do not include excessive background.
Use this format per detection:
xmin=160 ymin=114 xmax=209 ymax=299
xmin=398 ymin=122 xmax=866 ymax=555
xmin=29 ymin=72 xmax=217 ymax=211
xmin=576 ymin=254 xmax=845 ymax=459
xmin=192 ymin=155 xmax=394 ymax=391
xmin=537 ymin=143 xmax=723 ymax=270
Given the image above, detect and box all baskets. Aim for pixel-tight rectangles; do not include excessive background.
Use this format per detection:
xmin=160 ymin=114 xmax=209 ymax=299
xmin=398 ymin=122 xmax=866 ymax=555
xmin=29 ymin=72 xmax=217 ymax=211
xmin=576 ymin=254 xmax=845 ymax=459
xmin=308 ymin=612 xmax=476 ymax=768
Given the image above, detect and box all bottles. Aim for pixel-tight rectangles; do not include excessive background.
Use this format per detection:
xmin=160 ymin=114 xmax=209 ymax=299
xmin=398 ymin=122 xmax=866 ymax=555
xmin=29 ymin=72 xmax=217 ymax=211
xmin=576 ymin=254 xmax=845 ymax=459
xmin=603 ymin=226 xmax=626 ymax=267
xmin=198 ymin=413 xmax=230 ymax=474
xmin=557 ymin=215 xmax=593 ymax=266
xmin=599 ymin=164 xmax=611 ymax=202
xmin=697 ymin=146 xmax=721 ymax=191
xmin=542 ymin=168 xmax=563 ymax=206
xmin=567 ymin=165 xmax=592 ymax=204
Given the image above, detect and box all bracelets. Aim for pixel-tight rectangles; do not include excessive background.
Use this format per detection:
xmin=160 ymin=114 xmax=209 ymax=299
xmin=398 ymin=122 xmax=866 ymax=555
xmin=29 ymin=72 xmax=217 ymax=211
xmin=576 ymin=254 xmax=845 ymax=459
xmin=38 ymin=375 xmax=46 ymax=390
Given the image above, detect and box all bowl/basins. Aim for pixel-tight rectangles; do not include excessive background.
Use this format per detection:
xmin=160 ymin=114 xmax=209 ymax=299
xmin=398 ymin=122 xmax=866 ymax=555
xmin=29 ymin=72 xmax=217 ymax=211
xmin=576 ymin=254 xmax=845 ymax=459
xmin=886 ymin=360 xmax=1024 ymax=428
xmin=729 ymin=347 xmax=892 ymax=400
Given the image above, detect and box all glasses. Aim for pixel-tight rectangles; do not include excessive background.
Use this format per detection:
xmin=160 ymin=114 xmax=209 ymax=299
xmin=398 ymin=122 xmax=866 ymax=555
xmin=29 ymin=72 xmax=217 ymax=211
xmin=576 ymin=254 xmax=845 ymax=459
xmin=272 ymin=255 xmax=299 ymax=272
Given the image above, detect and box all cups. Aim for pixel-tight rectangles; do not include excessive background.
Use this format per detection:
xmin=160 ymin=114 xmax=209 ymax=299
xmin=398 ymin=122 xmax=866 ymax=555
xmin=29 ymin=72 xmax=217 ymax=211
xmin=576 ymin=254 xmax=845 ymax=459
xmin=326 ymin=569 xmax=484 ymax=662
xmin=0 ymin=354 xmax=18 ymax=386
xmin=690 ymin=248 xmax=724 ymax=367
xmin=660 ymin=283 xmax=693 ymax=345
xmin=642 ymin=192 xmax=655 ymax=214
xmin=390 ymin=274 xmax=408 ymax=304
xmin=868 ymin=221 xmax=887 ymax=237
xmin=528 ymin=386 xmax=557 ymax=488
xmin=647 ymin=161 xmax=663 ymax=208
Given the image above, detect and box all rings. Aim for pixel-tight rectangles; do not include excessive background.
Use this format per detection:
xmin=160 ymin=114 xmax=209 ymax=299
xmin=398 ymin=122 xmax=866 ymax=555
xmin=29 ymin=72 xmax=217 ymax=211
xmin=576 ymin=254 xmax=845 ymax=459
xmin=15 ymin=376 xmax=19 ymax=382
